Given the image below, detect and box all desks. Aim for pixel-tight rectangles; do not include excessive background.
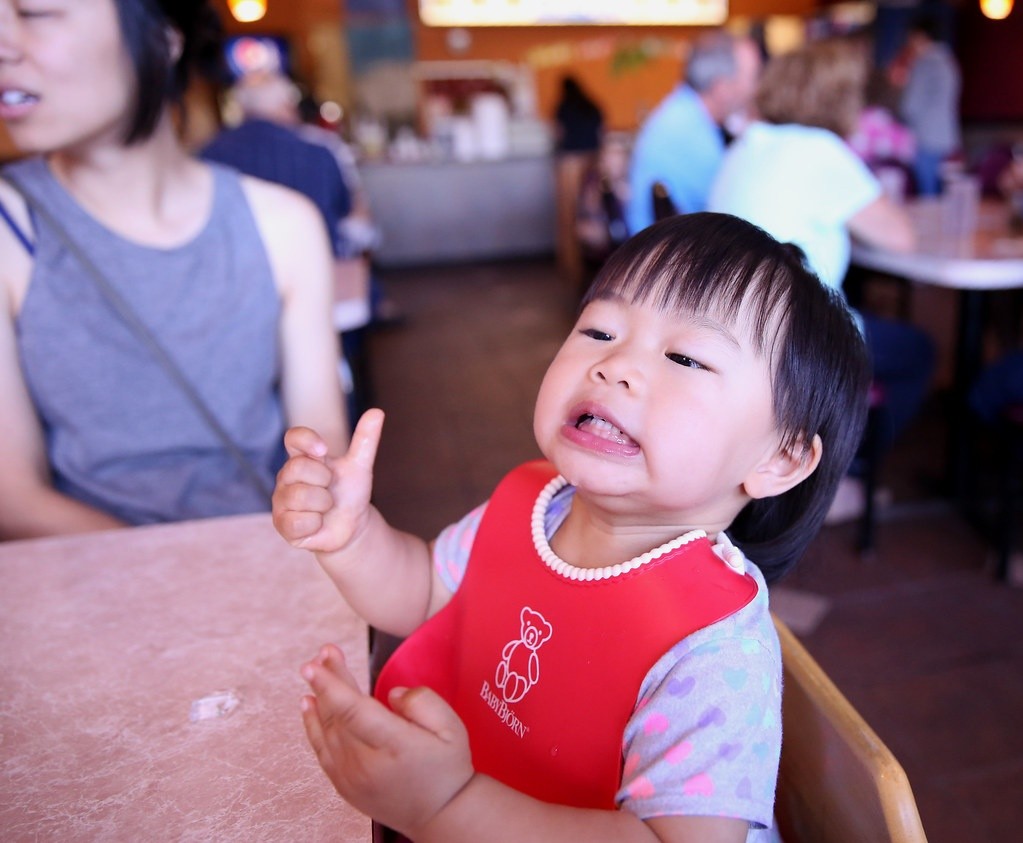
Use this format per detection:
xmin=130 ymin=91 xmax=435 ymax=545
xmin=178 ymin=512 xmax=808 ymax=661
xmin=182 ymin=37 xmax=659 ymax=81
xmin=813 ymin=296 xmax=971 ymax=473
xmin=0 ymin=513 xmax=373 ymax=843
xmin=851 ymin=238 xmax=1023 ymax=550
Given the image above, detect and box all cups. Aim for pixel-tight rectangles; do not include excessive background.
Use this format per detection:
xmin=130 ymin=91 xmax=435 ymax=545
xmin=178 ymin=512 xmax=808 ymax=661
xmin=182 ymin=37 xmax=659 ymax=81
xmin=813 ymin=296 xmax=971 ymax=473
xmin=940 ymin=174 xmax=982 ymax=243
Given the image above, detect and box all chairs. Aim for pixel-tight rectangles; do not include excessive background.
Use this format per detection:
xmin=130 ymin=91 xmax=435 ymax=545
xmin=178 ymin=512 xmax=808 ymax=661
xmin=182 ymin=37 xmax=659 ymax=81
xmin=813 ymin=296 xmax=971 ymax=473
xmin=770 ymin=612 xmax=928 ymax=843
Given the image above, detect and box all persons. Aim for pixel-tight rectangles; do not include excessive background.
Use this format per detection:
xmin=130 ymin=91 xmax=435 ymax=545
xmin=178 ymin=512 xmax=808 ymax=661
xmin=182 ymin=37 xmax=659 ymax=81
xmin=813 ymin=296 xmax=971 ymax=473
xmin=554 ymin=76 xmax=604 ymax=152
xmin=199 ymin=71 xmax=381 ymax=352
xmin=897 ymin=19 xmax=962 ymax=196
xmin=705 ymin=39 xmax=941 ymax=514
xmin=0 ymin=0 xmax=350 ymax=539
xmin=624 ymin=28 xmax=763 ymax=235
xmin=271 ymin=211 xmax=873 ymax=843
